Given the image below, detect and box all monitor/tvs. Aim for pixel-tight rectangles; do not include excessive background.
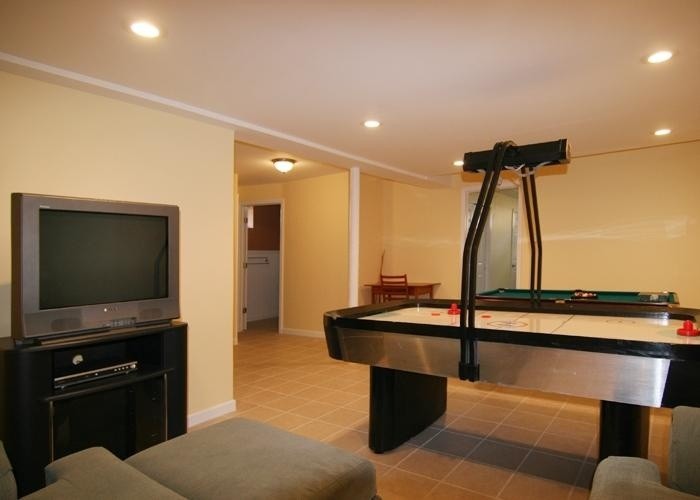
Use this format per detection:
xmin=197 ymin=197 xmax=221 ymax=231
xmin=10 ymin=192 xmax=181 ymax=346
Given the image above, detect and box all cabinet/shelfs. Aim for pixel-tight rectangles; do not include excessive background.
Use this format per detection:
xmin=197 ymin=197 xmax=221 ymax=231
xmin=0 ymin=317 xmax=189 ymax=499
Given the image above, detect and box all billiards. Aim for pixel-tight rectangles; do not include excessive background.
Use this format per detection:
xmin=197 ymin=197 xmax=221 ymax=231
xmin=574 ymin=291 xmax=593 ymax=297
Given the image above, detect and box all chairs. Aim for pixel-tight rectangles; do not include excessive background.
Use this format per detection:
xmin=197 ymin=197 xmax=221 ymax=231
xmin=585 ymin=406 xmax=700 ymax=500
xmin=379 ymin=274 xmax=409 ymax=303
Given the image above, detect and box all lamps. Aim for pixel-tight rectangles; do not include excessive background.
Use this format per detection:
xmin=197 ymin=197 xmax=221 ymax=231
xmin=271 ymin=158 xmax=296 ymax=174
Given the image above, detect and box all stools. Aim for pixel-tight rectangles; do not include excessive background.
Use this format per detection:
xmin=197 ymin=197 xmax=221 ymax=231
xmin=126 ymin=414 xmax=376 ymax=500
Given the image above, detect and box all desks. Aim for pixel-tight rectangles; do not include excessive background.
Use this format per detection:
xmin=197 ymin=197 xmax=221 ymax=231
xmin=364 ymin=281 xmax=442 ymax=303
xmin=477 ymin=288 xmax=680 ymax=313
